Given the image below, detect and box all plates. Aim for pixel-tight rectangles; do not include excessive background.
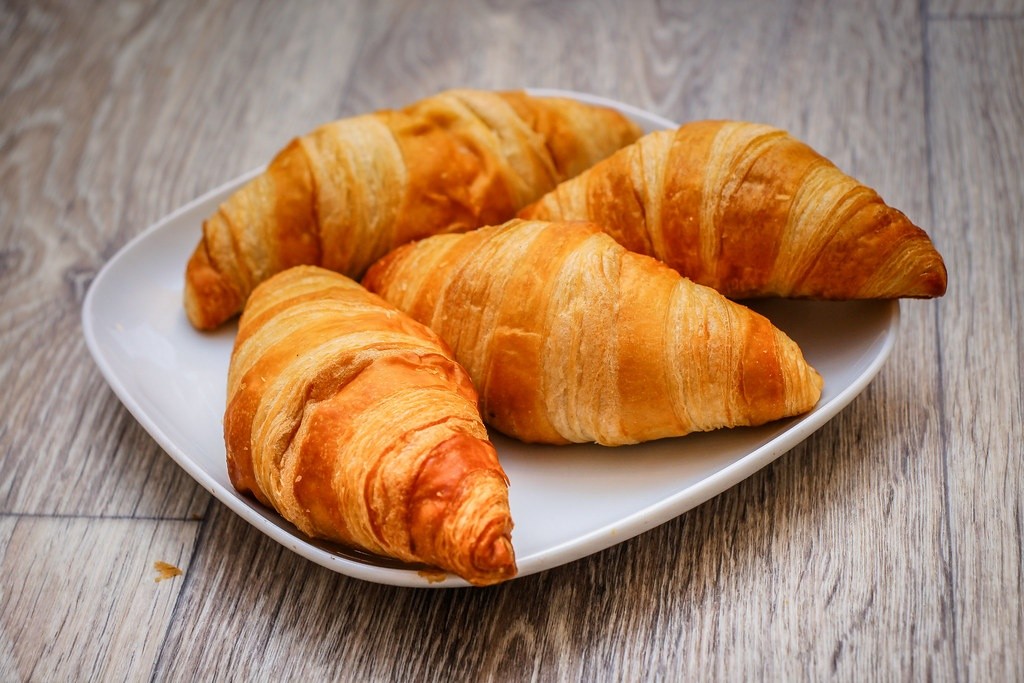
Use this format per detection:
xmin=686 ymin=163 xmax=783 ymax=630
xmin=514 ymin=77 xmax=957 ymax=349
xmin=82 ymin=87 xmax=901 ymax=589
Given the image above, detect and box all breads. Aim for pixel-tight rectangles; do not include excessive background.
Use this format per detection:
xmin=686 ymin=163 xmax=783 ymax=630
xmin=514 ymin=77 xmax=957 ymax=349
xmin=510 ymin=119 xmax=947 ymax=304
xmin=358 ymin=215 xmax=825 ymax=444
xmin=222 ymin=265 xmax=519 ymax=587
xmin=182 ymin=89 xmax=651 ymax=331
